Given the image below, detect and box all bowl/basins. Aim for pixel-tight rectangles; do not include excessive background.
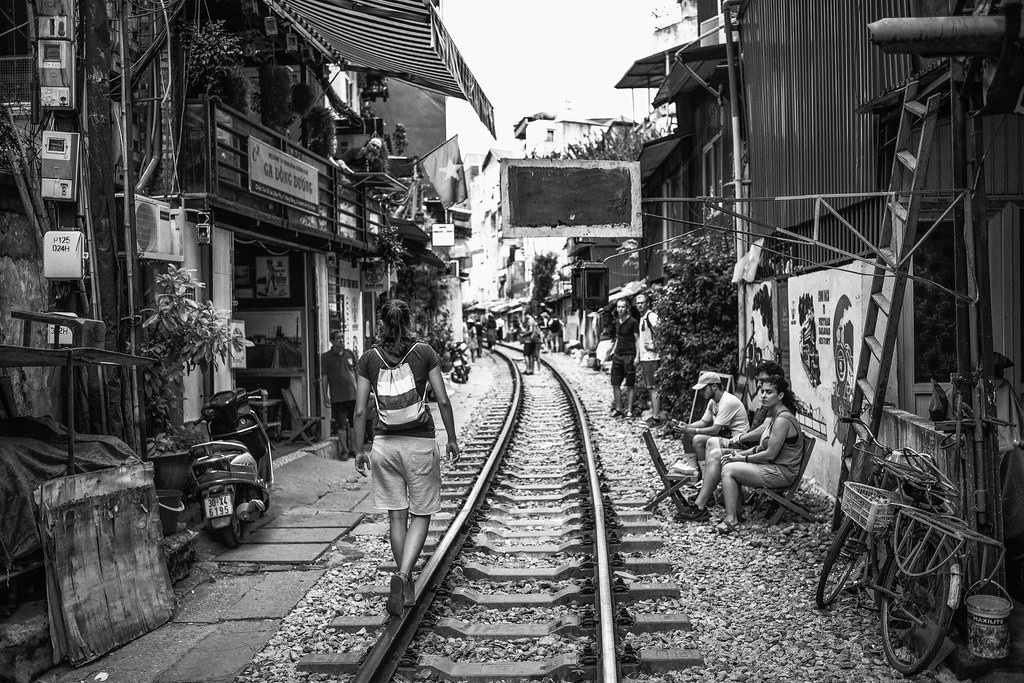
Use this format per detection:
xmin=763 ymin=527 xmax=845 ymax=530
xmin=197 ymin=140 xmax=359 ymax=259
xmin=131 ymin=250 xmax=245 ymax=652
xmin=672 ymin=466 xmax=696 ymax=474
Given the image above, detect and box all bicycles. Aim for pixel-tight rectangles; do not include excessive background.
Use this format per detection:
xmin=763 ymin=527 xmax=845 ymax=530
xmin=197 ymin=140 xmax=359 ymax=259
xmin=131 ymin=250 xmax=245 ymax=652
xmin=815 ymin=416 xmax=963 ymax=674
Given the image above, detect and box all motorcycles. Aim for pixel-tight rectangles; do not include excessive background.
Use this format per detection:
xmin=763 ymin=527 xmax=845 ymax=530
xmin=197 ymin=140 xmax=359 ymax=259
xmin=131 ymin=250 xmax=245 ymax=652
xmin=445 ymin=340 xmax=472 ymax=384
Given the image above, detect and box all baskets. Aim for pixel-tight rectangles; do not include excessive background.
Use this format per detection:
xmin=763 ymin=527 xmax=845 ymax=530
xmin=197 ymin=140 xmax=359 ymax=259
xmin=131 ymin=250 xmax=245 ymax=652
xmin=841 ymin=480 xmax=894 ymax=533
xmin=848 ymin=439 xmax=897 ymax=489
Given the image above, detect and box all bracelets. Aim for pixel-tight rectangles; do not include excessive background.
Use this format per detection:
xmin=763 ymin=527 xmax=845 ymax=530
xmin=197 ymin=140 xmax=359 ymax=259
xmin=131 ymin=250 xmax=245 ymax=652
xmin=745 ymin=454 xmax=749 ymax=462
xmin=696 ymin=427 xmax=699 ymax=434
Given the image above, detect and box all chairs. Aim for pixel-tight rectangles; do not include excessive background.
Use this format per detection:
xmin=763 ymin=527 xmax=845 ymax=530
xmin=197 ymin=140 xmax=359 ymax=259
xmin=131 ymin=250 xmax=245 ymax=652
xmin=282 ymin=388 xmax=325 ymax=445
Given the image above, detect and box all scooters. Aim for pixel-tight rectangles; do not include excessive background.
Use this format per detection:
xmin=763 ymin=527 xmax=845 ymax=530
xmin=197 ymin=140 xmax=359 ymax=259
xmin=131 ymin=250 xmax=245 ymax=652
xmin=187 ymin=389 xmax=274 ymax=549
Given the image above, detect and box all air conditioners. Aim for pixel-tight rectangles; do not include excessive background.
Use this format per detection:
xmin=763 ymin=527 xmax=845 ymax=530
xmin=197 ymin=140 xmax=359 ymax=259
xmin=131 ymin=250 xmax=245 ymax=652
xmin=115 ymin=193 xmax=171 ymax=255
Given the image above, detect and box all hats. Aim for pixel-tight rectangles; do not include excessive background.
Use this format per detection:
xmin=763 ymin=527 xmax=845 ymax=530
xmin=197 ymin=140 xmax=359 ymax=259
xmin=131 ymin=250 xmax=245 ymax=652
xmin=692 ymin=372 xmax=721 ymax=389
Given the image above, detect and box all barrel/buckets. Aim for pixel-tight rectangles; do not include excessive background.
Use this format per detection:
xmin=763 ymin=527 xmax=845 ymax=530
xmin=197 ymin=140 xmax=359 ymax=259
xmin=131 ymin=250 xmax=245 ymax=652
xmin=964 ymin=579 xmax=1013 ymax=659
xmin=155 ymin=490 xmax=185 ymax=536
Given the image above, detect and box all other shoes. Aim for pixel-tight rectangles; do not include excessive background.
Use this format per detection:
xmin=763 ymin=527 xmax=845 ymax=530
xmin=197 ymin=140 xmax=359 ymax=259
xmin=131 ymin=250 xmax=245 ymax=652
xmin=403 ymin=585 xmax=416 ymax=605
xmin=521 ymin=370 xmax=533 ymax=375
xmin=385 ymin=575 xmax=403 ymax=616
xmin=610 ymin=410 xmax=623 ymax=418
xmin=674 ymin=501 xmax=709 ymax=521
xmin=687 ymin=479 xmax=704 ymax=490
xmin=688 ymin=490 xmax=716 ymax=506
xmin=646 ymin=416 xmax=661 ymax=427
xmin=722 ymin=510 xmax=747 ymax=522
xmin=625 ymin=411 xmax=635 ymax=420
xmin=715 ymin=519 xmax=738 ymax=532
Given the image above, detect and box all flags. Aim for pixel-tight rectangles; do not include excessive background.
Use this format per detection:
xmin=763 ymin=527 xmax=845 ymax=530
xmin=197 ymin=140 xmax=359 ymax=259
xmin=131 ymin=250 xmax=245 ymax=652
xmin=422 ymin=133 xmax=469 ymax=209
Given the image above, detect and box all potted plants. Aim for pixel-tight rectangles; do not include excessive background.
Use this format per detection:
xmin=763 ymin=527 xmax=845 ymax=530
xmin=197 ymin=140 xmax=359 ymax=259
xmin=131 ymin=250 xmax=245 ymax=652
xmin=135 ymin=264 xmax=256 ymax=491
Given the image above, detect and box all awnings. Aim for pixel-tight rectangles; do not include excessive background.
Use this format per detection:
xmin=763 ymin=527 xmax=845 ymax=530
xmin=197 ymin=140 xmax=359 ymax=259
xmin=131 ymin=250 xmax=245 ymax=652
xmin=265 ymin=0 xmax=497 ymax=141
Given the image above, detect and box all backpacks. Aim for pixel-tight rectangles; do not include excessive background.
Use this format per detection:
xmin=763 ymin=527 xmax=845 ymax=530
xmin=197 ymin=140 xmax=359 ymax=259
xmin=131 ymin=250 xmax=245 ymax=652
xmin=550 ymin=318 xmax=559 ymax=333
xmin=366 ymin=343 xmax=426 ymax=425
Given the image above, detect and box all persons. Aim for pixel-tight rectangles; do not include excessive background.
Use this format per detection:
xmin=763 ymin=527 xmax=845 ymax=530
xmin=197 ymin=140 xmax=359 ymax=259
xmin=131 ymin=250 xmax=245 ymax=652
xmin=674 ymin=359 xmax=797 ymax=522
xmin=672 ymin=371 xmax=751 ymax=492
xmin=321 ymin=330 xmax=359 ymax=461
xmin=512 ymin=303 xmax=564 ymax=375
xmin=633 ymin=294 xmax=661 ymax=428
xmin=708 ymin=375 xmax=804 ymax=534
xmin=336 ymin=137 xmax=385 ymax=174
xmin=353 ymin=299 xmax=459 ymax=616
xmin=462 ymin=310 xmax=504 ymax=357
xmin=610 ymin=298 xmax=639 ymax=417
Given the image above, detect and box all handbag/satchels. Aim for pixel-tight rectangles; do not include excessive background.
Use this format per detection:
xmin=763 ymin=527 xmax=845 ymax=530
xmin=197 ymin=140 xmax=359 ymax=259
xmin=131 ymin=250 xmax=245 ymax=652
xmin=528 ymin=318 xmax=540 ymax=340
